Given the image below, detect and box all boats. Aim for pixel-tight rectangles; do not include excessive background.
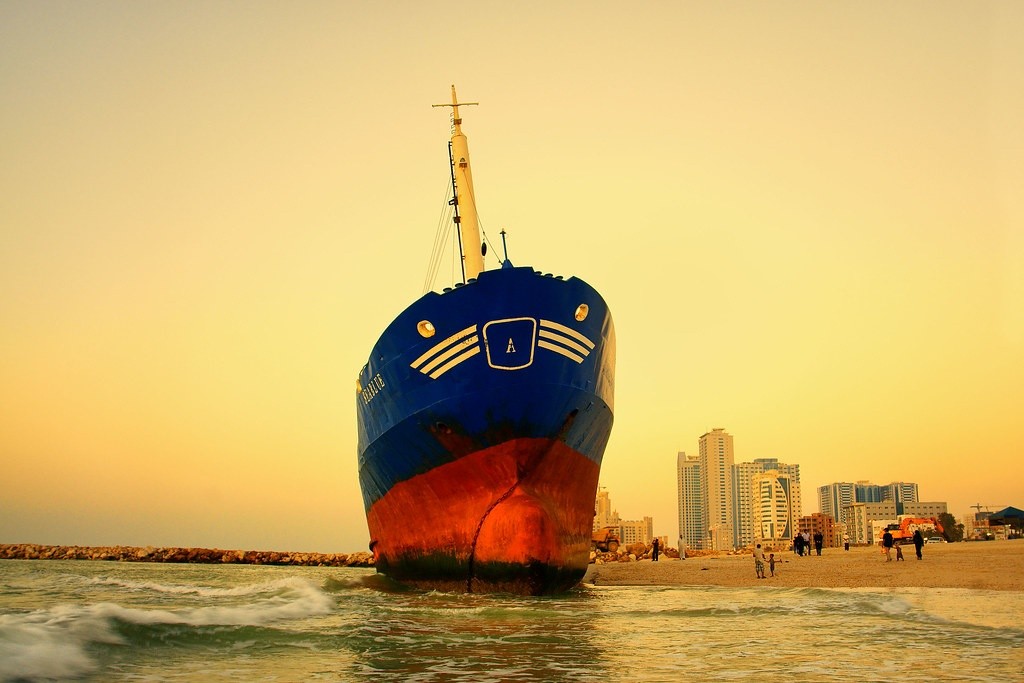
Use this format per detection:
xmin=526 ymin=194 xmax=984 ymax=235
xmin=352 ymin=81 xmax=618 ymax=596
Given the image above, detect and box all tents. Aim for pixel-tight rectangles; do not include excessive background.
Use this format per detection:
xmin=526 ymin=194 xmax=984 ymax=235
xmin=989 ymin=506 xmax=1024 ymax=541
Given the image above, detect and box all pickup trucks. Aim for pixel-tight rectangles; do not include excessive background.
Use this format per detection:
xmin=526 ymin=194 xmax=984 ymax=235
xmin=926 ymin=537 xmax=947 ymax=544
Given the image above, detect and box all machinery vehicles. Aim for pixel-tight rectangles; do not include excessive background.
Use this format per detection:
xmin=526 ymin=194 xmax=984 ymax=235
xmin=877 ymin=516 xmax=954 ymax=547
xmin=592 ymin=525 xmax=621 ymax=551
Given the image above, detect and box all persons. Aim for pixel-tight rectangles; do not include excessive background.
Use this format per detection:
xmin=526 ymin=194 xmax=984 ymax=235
xmin=793 ymin=529 xmax=812 ymax=556
xmin=813 ymin=530 xmax=823 ymax=556
xmin=882 ymin=528 xmax=904 ymax=562
xmin=766 ymin=554 xmax=775 ymax=577
xmin=678 ymin=535 xmax=686 ymax=560
xmin=843 ymin=533 xmax=849 ymax=551
xmin=652 ymin=539 xmax=658 ymax=561
xmin=913 ymin=530 xmax=924 ymax=560
xmin=753 ymin=544 xmax=766 ymax=578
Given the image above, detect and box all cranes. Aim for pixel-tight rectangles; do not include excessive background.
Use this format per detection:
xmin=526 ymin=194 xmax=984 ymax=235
xmin=969 ymin=502 xmax=1007 ymax=512
